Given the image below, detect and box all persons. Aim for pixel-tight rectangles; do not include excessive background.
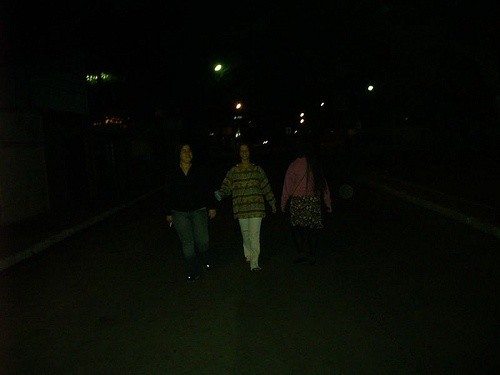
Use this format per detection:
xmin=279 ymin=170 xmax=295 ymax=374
xmin=214 ymin=142 xmax=277 ymax=272
xmin=281 ymin=139 xmax=334 ymax=263
xmin=166 ymin=141 xmax=217 ymax=283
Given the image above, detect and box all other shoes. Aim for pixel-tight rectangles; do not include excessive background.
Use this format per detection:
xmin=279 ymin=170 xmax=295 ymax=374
xmin=245 ymin=259 xmax=250 ymax=268
xmin=250 ymin=267 xmax=265 ymax=273
xmin=309 ymin=257 xmax=317 ymax=266
xmin=291 ymin=256 xmax=304 ymax=262
